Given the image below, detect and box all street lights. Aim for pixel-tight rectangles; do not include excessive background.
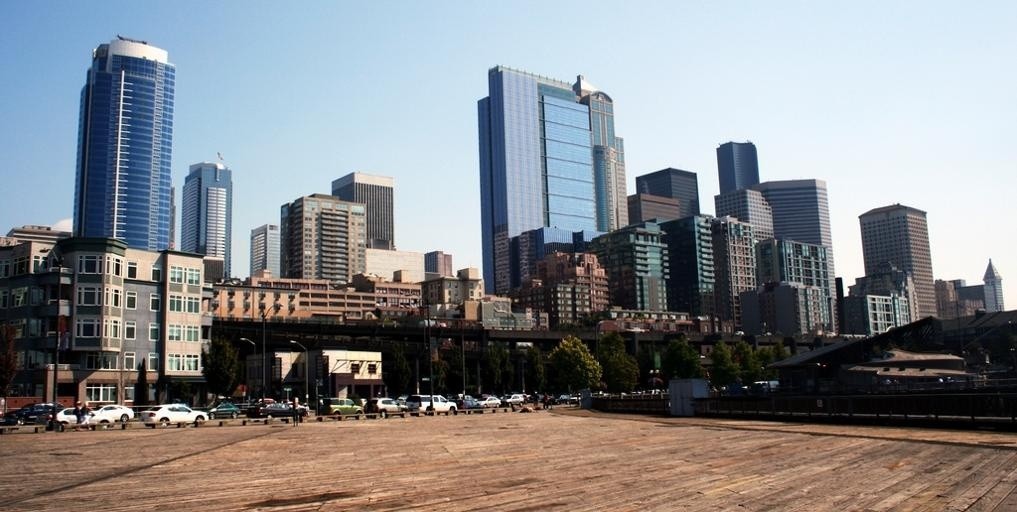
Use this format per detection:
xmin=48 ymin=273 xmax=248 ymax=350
xmin=239 ymin=337 xmax=259 ymax=403
xmin=288 ymin=339 xmax=310 ymax=408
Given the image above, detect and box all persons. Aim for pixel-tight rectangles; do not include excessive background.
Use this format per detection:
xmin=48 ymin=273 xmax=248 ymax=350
xmin=293 ymin=396 xmax=300 ymax=427
xmin=534 ymin=391 xmax=551 ymax=410
xmin=76 ymin=400 xmax=92 ymax=431
